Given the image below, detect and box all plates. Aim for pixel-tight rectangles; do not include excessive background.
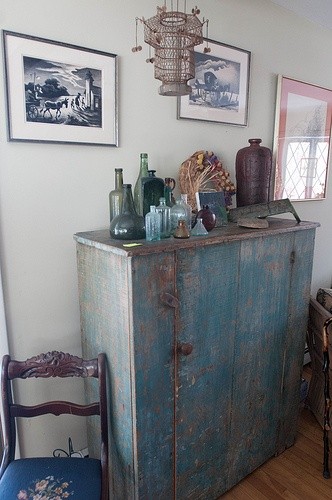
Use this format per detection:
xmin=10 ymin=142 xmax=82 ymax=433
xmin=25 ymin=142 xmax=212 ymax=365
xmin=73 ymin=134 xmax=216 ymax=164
xmin=179 ymin=149 xmax=235 ymax=214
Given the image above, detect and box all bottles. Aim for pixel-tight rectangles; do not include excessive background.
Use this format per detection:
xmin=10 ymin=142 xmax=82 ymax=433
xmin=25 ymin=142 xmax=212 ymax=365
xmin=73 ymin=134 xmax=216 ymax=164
xmin=133 ymin=153 xmax=148 ymax=219
xmin=145 ymin=205 xmax=161 ymax=241
xmin=171 ymin=200 xmax=187 ymax=235
xmin=191 ymin=218 xmax=208 ymax=236
xmin=235 ymin=139 xmax=272 ymax=207
xmin=196 ymin=204 xmax=216 ymax=232
xmin=141 ymin=170 xmax=165 ymax=226
xmin=180 ymin=195 xmax=192 ymax=238
xmin=108 ymin=168 xmax=124 ymax=222
xmin=174 ymin=220 xmax=189 ymax=238
xmin=163 ymin=177 xmax=175 ymax=206
xmin=110 ymin=183 xmax=146 ymax=239
xmin=157 ymin=197 xmax=171 ymax=239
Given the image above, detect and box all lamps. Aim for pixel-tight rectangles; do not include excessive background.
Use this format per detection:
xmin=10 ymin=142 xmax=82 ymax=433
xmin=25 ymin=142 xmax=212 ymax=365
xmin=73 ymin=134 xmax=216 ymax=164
xmin=130 ymin=0 xmax=211 ymax=97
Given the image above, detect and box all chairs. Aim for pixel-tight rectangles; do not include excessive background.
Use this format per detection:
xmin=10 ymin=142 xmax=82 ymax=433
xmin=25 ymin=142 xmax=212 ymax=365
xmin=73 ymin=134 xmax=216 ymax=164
xmin=305 ymin=285 xmax=332 ymax=479
xmin=0 ymin=350 xmax=111 ymax=500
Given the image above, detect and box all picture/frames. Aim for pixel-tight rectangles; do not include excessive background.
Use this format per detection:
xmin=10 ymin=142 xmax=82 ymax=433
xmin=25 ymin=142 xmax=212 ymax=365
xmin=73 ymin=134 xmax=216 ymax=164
xmin=177 ymin=30 xmax=251 ymax=129
xmin=0 ymin=28 xmax=119 ymax=147
xmin=270 ymin=73 xmax=332 ymax=203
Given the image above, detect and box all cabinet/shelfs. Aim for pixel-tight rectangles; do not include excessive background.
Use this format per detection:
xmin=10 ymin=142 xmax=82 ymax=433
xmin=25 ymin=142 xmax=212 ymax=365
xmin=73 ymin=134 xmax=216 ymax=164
xmin=70 ymin=215 xmax=322 ymax=500
